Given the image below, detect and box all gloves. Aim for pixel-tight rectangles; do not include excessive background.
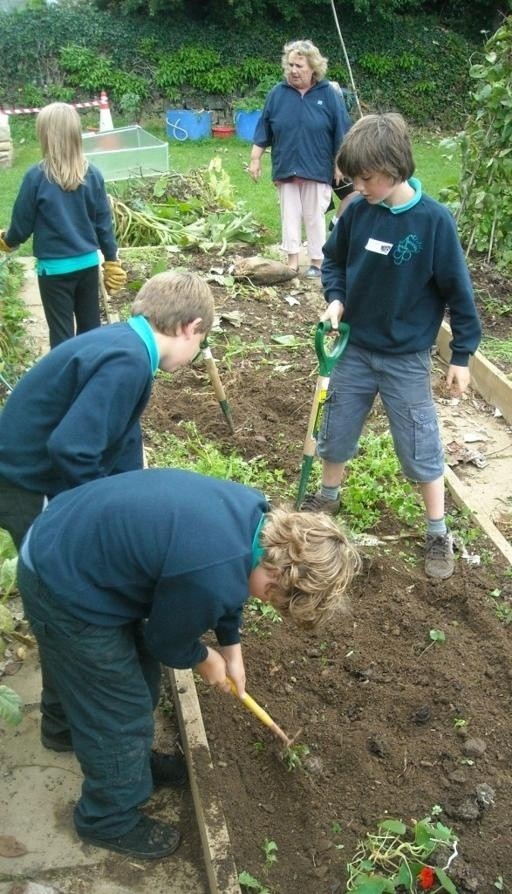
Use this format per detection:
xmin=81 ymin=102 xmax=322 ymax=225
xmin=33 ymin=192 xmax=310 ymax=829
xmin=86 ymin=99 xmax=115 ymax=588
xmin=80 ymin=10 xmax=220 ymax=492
xmin=102 ymin=259 xmax=127 ymax=297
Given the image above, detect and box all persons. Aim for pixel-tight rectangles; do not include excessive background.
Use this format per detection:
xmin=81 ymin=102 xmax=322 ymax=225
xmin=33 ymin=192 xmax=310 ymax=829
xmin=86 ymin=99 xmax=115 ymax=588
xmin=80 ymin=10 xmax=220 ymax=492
xmin=0 ymin=101 xmax=129 ymax=349
xmin=297 ymin=114 xmax=483 ymax=582
xmin=0 ymin=271 xmax=216 ymax=752
xmin=248 ymin=41 xmax=353 ymax=277
xmin=312 ymin=58 xmax=362 ymax=232
xmin=16 ymin=466 xmax=364 ymax=858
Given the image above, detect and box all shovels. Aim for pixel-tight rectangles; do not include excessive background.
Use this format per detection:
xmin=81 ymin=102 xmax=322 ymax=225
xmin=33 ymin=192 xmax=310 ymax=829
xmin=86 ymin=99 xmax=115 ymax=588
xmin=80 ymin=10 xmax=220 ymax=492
xmin=224 ymin=677 xmax=307 ymax=772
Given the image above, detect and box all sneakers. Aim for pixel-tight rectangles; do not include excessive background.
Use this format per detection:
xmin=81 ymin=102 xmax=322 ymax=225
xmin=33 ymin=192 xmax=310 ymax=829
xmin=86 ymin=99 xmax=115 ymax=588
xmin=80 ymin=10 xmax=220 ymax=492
xmin=149 ymin=749 xmax=189 ymax=790
xmin=40 ymin=723 xmax=74 ymax=752
xmin=424 ymin=526 xmax=455 ymax=579
xmin=76 ymin=815 xmax=182 ymax=860
xmin=300 ymin=491 xmax=341 ymax=515
xmin=305 ymin=266 xmax=322 ymax=280
